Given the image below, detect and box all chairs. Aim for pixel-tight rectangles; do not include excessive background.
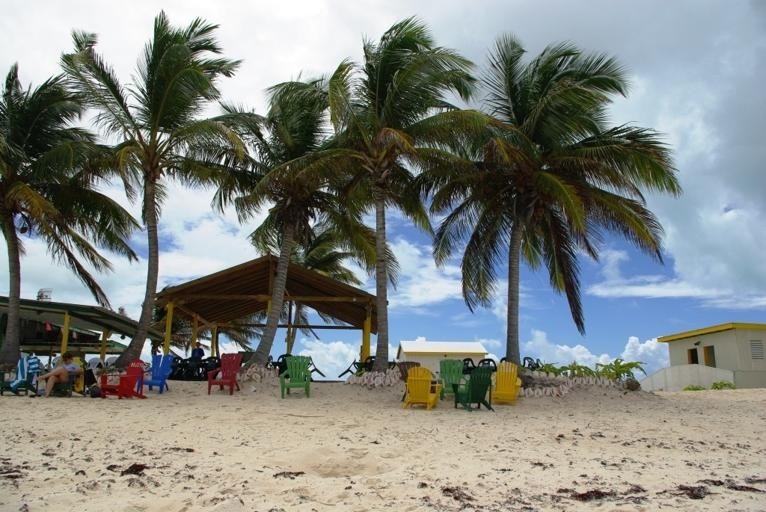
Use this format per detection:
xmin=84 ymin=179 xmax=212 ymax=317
xmin=136 ymin=354 xmax=173 ymax=395
xmin=206 ymin=353 xmax=243 ymax=396
xmin=169 ymin=354 xmax=220 ymax=382
xmin=396 ymin=357 xmax=539 ymax=413
xmin=10 ymin=355 xmax=150 ymax=399
xmin=279 ymin=356 xmax=311 ymax=399
xmin=266 ymin=354 xmax=325 ymax=380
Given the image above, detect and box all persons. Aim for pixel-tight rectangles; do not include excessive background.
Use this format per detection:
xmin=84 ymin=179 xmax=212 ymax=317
xmin=36 ymin=351 xmax=86 ymax=398
xmin=190 ymin=341 xmax=205 ymax=380
